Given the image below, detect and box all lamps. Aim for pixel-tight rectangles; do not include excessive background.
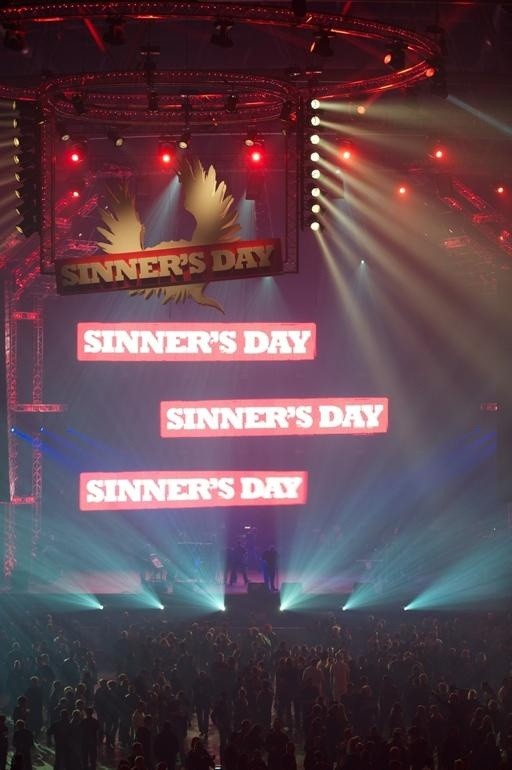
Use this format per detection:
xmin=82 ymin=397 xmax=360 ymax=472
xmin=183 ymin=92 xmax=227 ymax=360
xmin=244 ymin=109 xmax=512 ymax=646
xmin=304 ymin=94 xmax=324 ymax=233
xmin=50 ymin=90 xmax=295 ymax=148
xmin=10 ymin=99 xmax=43 ymax=238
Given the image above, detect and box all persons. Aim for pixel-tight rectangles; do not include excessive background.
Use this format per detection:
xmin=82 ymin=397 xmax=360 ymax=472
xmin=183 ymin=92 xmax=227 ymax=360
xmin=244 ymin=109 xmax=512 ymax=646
xmin=0 ymin=531 xmax=512 ymax=769
